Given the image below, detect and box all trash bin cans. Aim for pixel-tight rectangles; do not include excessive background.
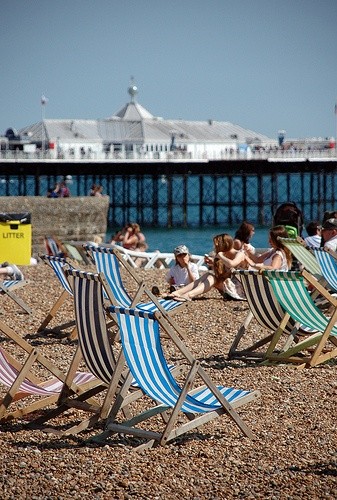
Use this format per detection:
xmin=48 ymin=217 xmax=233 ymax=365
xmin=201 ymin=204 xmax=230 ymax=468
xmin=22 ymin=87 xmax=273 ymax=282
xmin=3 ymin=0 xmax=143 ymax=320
xmin=0 ymin=211 xmax=33 ymax=266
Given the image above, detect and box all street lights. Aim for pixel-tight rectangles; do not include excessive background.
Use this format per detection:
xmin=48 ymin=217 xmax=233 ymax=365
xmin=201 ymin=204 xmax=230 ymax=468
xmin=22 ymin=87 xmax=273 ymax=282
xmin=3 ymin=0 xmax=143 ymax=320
xmin=40 ymin=94 xmax=49 ymax=158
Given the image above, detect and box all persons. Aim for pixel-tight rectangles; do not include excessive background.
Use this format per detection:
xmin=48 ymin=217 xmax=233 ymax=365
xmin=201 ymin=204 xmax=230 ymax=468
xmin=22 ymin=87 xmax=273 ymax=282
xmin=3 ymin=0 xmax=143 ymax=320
xmin=48 ymin=183 xmax=146 ymax=252
xmin=166 ymin=211 xmax=337 ymax=301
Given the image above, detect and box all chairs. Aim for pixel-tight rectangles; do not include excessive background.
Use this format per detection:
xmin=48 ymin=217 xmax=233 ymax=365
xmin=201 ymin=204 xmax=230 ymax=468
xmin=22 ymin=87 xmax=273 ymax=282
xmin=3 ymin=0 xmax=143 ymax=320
xmin=0 ymin=236 xmax=337 ymax=453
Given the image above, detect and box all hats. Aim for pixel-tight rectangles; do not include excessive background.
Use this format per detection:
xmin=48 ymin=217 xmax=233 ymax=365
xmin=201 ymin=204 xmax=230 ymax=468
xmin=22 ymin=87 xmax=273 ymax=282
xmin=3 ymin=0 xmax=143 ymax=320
xmin=173 ymin=245 xmax=189 ymax=255
xmin=317 ymin=218 xmax=337 ymax=230
